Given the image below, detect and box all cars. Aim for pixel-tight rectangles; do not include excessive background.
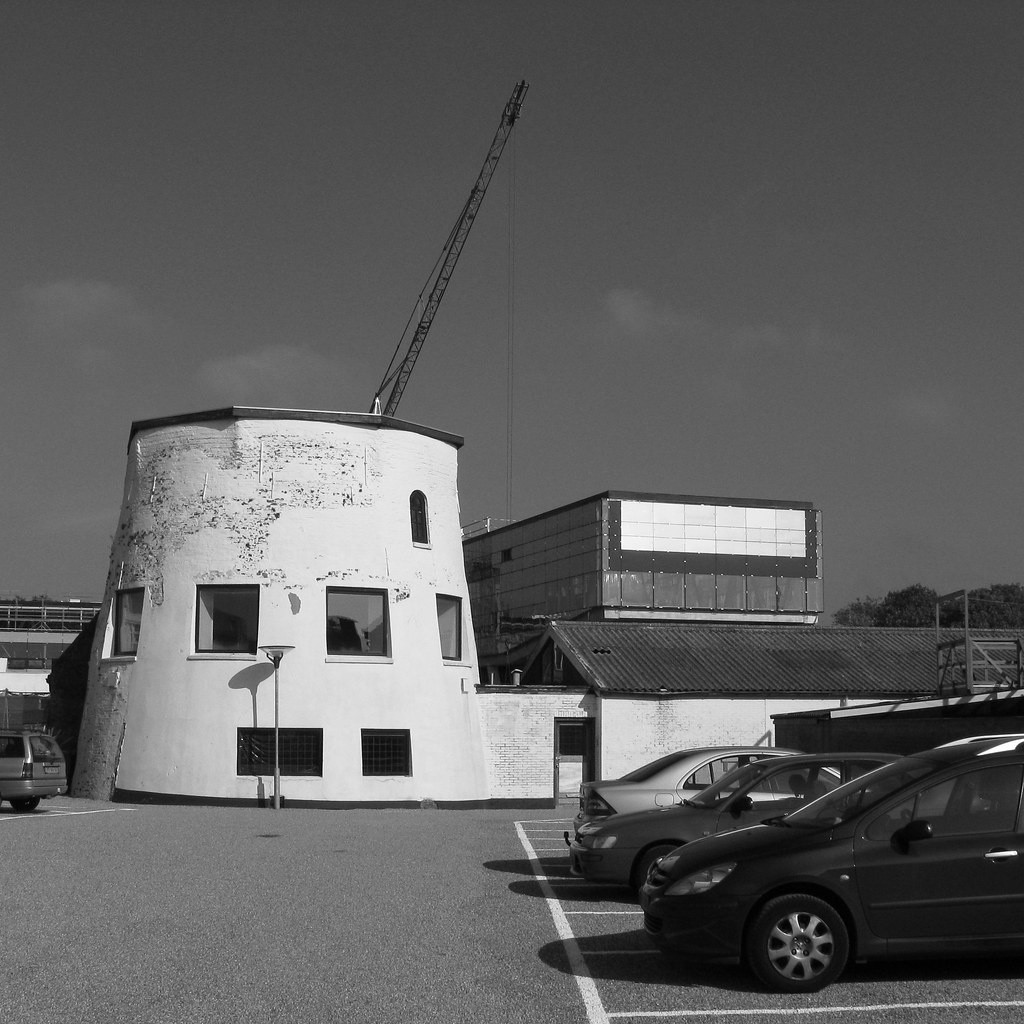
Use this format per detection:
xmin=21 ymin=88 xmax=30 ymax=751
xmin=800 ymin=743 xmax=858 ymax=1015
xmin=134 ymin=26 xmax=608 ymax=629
xmin=573 ymin=745 xmax=870 ymax=856
xmin=569 ymin=751 xmax=905 ymax=895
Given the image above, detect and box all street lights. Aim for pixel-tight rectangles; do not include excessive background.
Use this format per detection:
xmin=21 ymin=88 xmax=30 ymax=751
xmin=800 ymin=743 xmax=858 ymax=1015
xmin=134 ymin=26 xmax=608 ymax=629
xmin=258 ymin=643 xmax=295 ymax=808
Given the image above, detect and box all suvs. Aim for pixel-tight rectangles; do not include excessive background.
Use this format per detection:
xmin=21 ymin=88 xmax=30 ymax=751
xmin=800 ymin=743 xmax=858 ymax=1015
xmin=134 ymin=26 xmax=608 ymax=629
xmin=0 ymin=728 xmax=68 ymax=813
xmin=639 ymin=733 xmax=1024 ymax=995
